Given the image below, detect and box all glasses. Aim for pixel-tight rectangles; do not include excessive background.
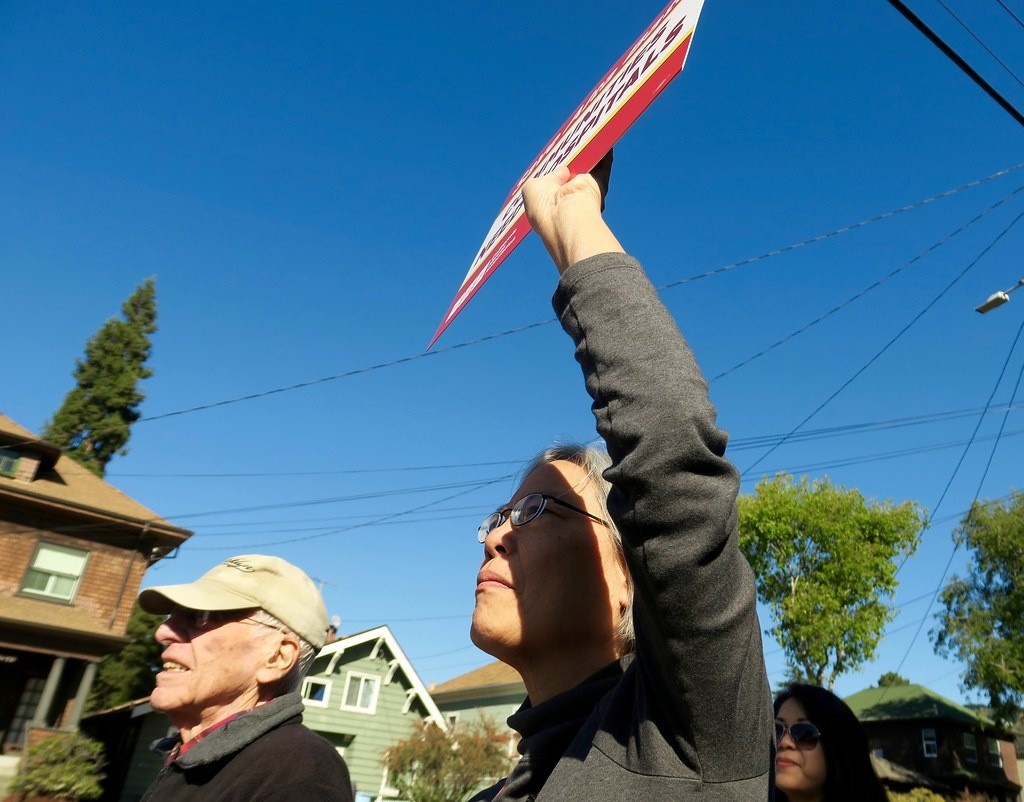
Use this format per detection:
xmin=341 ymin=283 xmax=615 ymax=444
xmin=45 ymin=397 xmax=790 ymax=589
xmin=478 ymin=492 xmax=612 ymax=544
xmin=775 ymin=722 xmax=822 ymax=751
xmin=173 ymin=607 xmax=286 ymax=637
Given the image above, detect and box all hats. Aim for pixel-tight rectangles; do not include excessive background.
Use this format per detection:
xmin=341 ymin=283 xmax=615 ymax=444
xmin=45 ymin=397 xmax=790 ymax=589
xmin=137 ymin=554 xmax=329 ymax=652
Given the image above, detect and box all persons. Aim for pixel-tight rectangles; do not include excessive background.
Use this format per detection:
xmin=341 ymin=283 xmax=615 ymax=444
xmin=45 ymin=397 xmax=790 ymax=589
xmin=470 ymin=150 xmax=774 ymax=802
xmin=772 ymin=682 xmax=892 ymax=802
xmin=137 ymin=554 xmax=355 ymax=802
